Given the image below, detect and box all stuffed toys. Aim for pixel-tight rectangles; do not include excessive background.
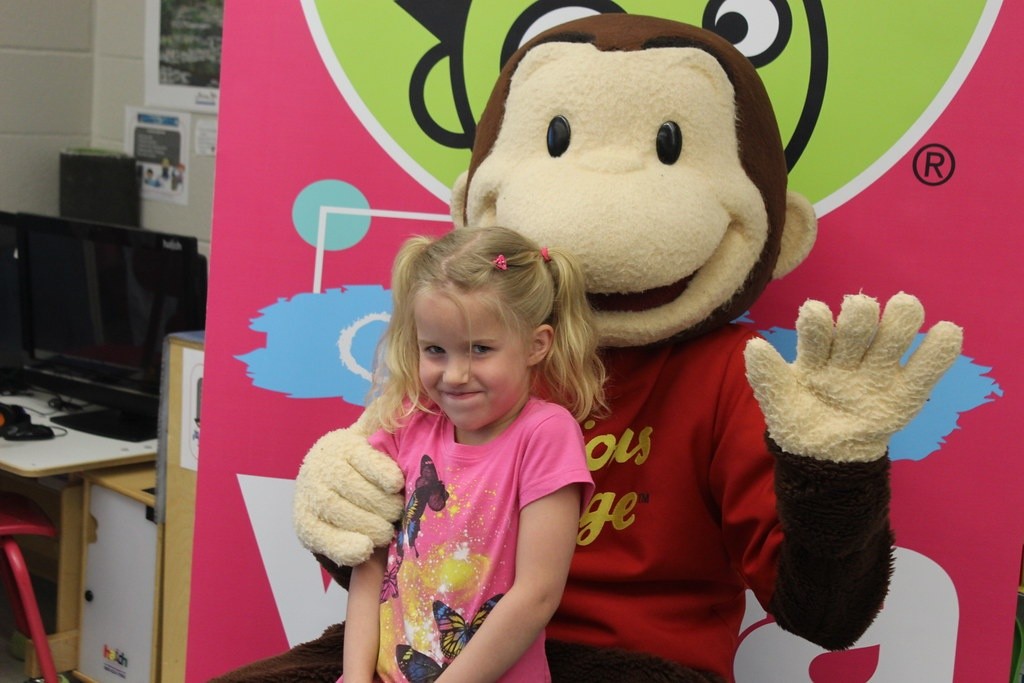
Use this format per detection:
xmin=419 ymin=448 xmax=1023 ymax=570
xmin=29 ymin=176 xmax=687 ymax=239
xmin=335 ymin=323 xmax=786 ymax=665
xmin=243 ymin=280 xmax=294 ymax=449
xmin=215 ymin=14 xmax=964 ymax=682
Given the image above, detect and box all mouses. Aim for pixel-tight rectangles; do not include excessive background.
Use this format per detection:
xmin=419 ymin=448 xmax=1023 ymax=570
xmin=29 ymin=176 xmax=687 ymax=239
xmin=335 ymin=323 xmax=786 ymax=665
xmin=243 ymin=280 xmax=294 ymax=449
xmin=4 ymin=423 xmax=54 ymax=441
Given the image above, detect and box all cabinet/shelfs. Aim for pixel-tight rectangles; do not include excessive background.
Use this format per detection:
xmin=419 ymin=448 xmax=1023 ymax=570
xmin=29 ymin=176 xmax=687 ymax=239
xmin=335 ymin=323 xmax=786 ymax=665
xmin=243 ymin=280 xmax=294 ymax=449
xmin=71 ymin=462 xmax=165 ymax=683
xmin=153 ymin=331 xmax=205 ymax=683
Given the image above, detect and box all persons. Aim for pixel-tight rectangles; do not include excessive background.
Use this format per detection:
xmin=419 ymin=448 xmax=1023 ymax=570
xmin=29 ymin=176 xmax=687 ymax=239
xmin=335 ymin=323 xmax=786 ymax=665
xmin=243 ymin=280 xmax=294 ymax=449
xmin=332 ymin=223 xmax=604 ymax=683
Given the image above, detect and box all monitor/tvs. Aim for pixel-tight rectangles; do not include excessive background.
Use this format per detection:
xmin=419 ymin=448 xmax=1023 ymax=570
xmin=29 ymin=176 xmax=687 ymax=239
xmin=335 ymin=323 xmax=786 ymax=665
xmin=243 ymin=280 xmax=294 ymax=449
xmin=0 ymin=211 xmax=207 ymax=442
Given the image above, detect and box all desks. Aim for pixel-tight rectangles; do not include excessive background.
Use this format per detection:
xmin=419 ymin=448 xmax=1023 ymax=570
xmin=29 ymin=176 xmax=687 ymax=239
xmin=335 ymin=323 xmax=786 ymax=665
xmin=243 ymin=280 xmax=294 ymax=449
xmin=1 ymin=331 xmax=157 ymax=683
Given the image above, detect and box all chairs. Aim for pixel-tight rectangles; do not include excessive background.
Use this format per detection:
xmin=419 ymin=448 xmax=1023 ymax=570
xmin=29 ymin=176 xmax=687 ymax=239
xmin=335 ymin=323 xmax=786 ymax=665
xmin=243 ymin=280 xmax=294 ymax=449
xmin=0 ymin=489 xmax=62 ymax=683
